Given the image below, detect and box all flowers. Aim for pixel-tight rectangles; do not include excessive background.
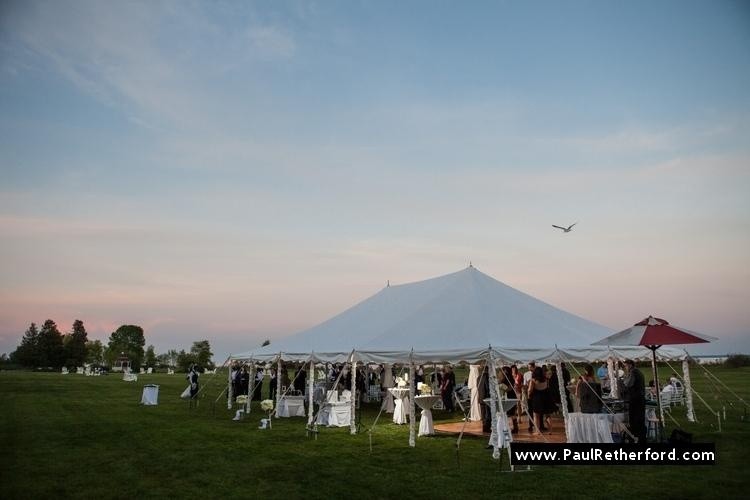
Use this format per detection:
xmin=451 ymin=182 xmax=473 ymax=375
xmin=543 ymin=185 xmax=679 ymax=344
xmin=260 ymin=398 xmax=274 ymax=412
xmin=235 ymin=394 xmax=248 ymax=405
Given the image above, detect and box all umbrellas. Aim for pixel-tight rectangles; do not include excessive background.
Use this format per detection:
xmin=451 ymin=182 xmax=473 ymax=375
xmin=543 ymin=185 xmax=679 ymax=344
xmin=590 ymin=313 xmax=718 ymax=427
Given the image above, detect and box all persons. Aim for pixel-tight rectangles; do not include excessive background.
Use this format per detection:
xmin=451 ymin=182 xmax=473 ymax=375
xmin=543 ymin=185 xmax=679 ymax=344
xmin=186 ymin=364 xmax=201 ymax=409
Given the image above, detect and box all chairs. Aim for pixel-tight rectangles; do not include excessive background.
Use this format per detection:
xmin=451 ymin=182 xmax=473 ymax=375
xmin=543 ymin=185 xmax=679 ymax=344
xmin=275 ymin=375 xmax=687 ymax=442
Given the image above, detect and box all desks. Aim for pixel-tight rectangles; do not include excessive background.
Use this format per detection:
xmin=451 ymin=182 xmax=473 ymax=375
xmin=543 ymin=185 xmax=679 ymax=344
xmin=143 ymin=382 xmax=160 ymax=407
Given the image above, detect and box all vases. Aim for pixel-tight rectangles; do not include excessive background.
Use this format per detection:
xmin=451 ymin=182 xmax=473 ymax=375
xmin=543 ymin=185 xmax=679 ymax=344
xmin=266 ymin=410 xmax=272 ymax=429
xmin=238 ymin=404 xmax=244 ymax=420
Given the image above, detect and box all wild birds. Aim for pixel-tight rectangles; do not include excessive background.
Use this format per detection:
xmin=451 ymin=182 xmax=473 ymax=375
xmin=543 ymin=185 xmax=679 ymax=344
xmin=550 ymin=222 xmax=577 ymax=233
